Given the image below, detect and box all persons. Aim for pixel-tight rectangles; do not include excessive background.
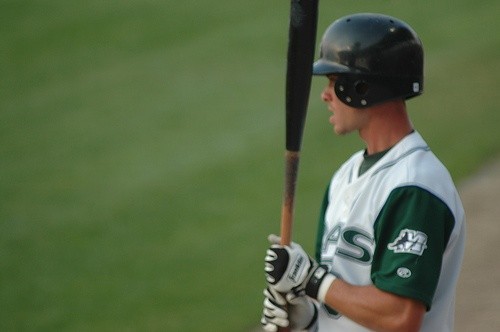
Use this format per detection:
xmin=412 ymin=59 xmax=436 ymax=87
xmin=261 ymin=13 xmax=468 ymax=332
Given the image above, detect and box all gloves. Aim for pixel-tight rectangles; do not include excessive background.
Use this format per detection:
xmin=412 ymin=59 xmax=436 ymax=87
xmin=258 ymin=288 xmax=320 ymax=332
xmin=262 ymin=233 xmax=337 ymax=307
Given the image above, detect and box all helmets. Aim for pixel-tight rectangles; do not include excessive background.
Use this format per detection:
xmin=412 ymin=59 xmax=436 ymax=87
xmin=311 ymin=13 xmax=426 ymax=109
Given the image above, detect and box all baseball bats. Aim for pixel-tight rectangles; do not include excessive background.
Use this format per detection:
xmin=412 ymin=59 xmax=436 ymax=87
xmin=277 ymin=0 xmax=319 ymax=332
